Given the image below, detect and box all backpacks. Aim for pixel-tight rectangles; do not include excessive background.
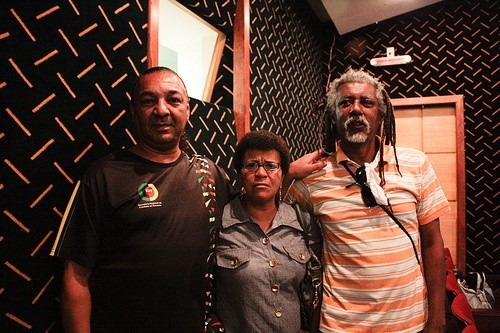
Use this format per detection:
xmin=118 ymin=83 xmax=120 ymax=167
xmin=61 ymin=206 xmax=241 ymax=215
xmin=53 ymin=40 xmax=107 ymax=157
xmin=452 ymin=268 xmax=495 ymax=309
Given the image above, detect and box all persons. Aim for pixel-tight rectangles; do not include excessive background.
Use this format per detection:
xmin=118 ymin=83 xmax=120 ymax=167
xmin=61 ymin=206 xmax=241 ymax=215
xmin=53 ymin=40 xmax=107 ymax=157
xmin=284 ymin=69 xmax=447 ymax=333
xmin=58 ymin=67 xmax=327 ymax=333
xmin=212 ymin=131 xmax=324 ymax=333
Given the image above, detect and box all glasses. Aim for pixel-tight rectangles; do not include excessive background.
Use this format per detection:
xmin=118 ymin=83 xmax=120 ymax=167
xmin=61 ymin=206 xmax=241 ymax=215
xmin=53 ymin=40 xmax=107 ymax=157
xmin=240 ymin=162 xmax=282 ymax=172
xmin=355 ymin=166 xmax=377 ymax=207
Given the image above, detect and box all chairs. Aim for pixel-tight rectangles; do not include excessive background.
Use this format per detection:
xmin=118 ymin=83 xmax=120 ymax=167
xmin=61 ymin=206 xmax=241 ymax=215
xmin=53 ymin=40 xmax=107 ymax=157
xmin=444 ymin=248 xmax=479 ymax=333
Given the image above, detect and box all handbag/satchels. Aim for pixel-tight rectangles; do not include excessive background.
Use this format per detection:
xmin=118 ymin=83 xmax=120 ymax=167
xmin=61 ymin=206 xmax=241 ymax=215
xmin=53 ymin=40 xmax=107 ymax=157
xmin=293 ymin=201 xmax=322 ymax=333
